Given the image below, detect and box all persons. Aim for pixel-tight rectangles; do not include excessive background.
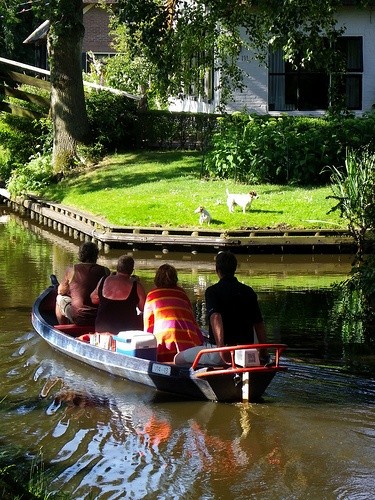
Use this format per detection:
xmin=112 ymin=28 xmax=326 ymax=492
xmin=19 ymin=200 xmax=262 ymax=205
xmin=90 ymin=254 xmax=147 ymax=335
xmin=144 ymin=265 xmax=205 ymax=362
xmin=173 ymin=252 xmax=269 ymax=366
xmin=55 ymin=242 xmax=110 ymax=327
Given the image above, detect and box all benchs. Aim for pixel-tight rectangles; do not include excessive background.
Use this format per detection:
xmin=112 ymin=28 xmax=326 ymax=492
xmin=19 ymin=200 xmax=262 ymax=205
xmin=55 ymin=324 xmax=95 ymax=335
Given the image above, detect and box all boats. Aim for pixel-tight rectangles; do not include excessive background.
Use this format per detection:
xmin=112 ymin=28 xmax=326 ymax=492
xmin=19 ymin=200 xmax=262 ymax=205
xmin=31 ymin=275 xmax=288 ymax=399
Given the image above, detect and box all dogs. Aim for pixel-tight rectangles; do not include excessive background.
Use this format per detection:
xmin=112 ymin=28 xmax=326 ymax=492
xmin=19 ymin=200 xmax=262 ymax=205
xmin=225 ymin=188 xmax=260 ymax=214
xmin=194 ymin=206 xmax=212 ymax=225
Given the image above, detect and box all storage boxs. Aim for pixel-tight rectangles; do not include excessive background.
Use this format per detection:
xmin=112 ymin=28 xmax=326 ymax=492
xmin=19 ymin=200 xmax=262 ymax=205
xmin=112 ymin=330 xmax=158 ymax=361
